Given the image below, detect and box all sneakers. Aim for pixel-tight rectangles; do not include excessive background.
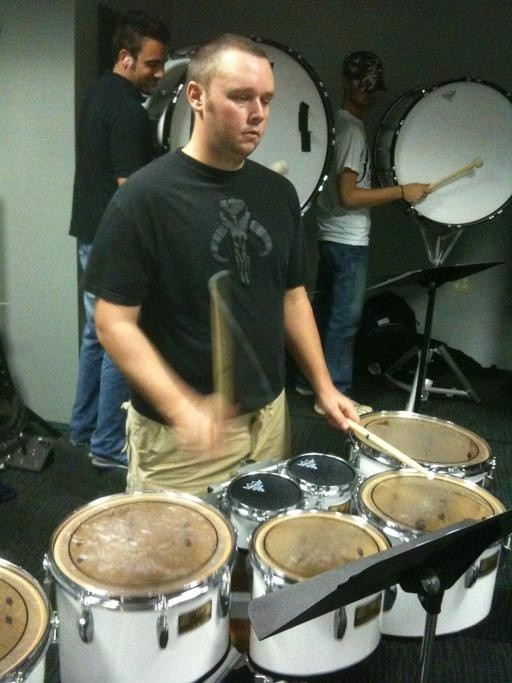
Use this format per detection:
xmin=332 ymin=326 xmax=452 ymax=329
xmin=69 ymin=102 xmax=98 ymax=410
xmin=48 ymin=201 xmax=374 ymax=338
xmin=314 ymin=396 xmax=372 ymax=417
xmin=92 ymin=440 xmax=128 ymax=470
xmin=296 ymin=385 xmax=316 ymax=396
xmin=71 ymin=437 xmax=91 ymax=446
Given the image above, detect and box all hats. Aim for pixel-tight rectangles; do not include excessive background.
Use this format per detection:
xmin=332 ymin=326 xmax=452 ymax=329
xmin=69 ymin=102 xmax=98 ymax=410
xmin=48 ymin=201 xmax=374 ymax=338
xmin=343 ymin=50 xmax=387 ymax=92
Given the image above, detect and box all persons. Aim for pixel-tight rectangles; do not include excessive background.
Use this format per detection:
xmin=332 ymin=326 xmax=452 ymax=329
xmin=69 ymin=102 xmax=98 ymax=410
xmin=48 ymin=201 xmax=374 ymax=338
xmin=68 ymin=16 xmax=169 ymax=460
xmin=296 ymin=49 xmax=433 ymax=416
xmin=93 ymin=33 xmax=372 ymax=493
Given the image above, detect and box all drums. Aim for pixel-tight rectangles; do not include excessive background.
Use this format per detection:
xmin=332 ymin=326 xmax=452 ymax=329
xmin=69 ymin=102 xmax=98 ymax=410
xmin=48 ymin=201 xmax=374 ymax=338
xmin=356 ymin=469 xmax=512 ymax=638
xmin=247 ymin=511 xmax=393 ymax=683
xmin=372 ymin=78 xmax=512 ymax=228
xmin=142 ymin=34 xmax=336 ymax=220
xmin=42 ymin=489 xmax=235 ymax=683
xmin=220 ymin=473 xmax=300 ymax=550
xmin=350 ymin=410 xmax=496 ymax=514
xmin=0 ymin=556 xmax=59 ymax=683
xmin=285 ymin=451 xmax=355 ymax=512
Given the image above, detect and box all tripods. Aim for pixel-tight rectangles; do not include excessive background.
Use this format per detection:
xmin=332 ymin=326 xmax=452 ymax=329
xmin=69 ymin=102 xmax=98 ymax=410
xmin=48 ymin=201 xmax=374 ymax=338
xmin=374 ymin=224 xmax=480 ymax=413
